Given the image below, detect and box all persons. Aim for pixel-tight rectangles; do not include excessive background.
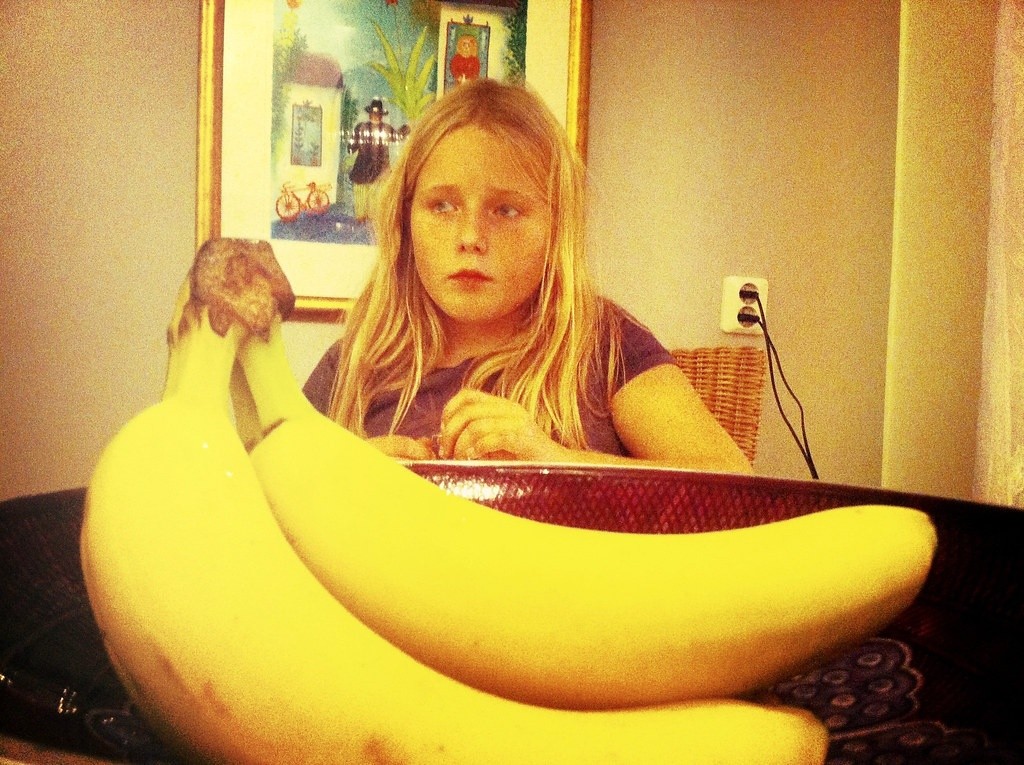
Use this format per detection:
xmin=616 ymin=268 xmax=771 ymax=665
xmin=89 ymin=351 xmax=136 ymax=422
xmin=303 ymin=79 xmax=756 ymax=478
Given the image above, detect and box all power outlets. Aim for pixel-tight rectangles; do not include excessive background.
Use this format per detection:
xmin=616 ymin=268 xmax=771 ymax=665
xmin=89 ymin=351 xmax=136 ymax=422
xmin=719 ymin=275 xmax=769 ymax=336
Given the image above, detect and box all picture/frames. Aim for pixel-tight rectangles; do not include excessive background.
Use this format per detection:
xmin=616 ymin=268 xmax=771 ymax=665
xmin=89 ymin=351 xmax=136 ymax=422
xmin=196 ymin=0 xmax=593 ymax=325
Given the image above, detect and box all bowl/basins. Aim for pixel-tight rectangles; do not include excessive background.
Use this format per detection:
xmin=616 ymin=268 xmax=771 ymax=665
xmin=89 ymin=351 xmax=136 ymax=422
xmin=0 ymin=460 xmax=1024 ymax=765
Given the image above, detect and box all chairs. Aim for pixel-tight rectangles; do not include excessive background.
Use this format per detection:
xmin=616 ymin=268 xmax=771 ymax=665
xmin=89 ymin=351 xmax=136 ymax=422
xmin=663 ymin=342 xmax=769 ymax=469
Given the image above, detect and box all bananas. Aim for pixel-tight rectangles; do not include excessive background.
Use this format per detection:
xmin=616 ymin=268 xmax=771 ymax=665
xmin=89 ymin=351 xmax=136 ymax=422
xmin=81 ymin=236 xmax=936 ymax=765
